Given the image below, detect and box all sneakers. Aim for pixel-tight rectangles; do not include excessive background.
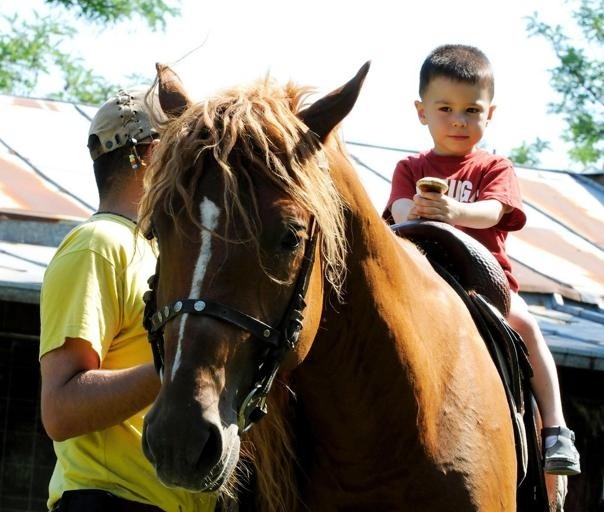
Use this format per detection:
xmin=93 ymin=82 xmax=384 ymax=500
xmin=540 ymin=425 xmax=581 ymax=475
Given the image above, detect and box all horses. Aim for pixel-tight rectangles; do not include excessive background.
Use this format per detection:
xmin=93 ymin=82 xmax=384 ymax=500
xmin=117 ymin=42 xmax=567 ymax=511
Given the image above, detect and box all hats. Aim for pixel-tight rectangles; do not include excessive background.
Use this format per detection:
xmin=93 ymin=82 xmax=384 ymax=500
xmin=89 ymin=91 xmax=166 ymax=160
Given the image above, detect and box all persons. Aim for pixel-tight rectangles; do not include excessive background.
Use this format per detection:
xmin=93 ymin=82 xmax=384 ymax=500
xmin=38 ymin=87 xmax=245 ymax=512
xmin=390 ymin=43 xmax=581 ymax=475
